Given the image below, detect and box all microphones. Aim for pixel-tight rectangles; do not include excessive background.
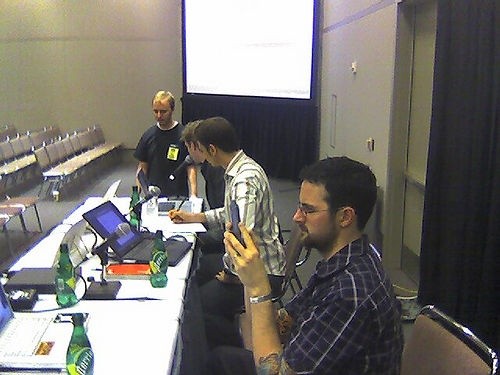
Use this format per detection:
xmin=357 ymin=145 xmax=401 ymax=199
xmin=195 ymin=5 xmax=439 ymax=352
xmin=127 ymin=187 xmax=161 ymax=215
xmin=169 ymin=155 xmax=191 ymax=180
xmin=83 ymin=223 xmax=131 ymax=261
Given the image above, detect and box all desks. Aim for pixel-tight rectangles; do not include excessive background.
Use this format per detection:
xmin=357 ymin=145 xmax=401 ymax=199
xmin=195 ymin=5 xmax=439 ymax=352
xmin=0 ymin=194 xmax=203 ymax=374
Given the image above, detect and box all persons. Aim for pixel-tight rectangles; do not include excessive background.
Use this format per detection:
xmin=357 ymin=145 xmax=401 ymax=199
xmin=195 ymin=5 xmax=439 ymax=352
xmin=168 ymin=116 xmax=400 ymax=375
xmin=132 ymin=90 xmax=197 ymax=198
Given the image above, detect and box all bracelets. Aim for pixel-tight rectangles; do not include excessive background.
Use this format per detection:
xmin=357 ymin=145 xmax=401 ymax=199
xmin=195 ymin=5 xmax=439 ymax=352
xmin=249 ymin=293 xmax=272 ymax=304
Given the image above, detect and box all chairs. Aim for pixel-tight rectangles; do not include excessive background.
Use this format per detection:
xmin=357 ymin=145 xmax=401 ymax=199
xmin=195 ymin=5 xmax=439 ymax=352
xmin=231 ymin=218 xmax=313 ymax=308
xmin=402 ymin=306 xmax=499 ymax=375
xmin=0 ymin=125 xmax=121 ymax=261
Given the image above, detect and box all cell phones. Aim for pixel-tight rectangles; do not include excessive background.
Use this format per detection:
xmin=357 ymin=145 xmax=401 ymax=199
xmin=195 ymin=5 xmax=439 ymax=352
xmin=230 ymin=201 xmax=242 ymax=241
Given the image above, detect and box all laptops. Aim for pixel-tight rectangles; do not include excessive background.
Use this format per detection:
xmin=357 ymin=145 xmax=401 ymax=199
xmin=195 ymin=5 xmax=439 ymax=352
xmin=0 ymin=281 xmax=90 ymax=368
xmin=136 ymin=167 xmax=192 ymax=216
xmin=82 ymin=200 xmax=194 ymax=267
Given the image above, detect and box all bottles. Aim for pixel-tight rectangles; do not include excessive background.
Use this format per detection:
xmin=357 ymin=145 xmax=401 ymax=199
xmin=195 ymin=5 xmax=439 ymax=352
xmin=149 ymin=230 xmax=168 ymax=288
xmin=54 ymin=243 xmax=78 ymax=307
xmin=129 ymin=186 xmax=142 ymax=227
xmin=66 ymin=312 xmax=94 ymax=375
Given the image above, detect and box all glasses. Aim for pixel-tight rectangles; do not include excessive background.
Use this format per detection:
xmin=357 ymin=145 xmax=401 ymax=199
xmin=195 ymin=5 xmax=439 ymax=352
xmin=296 ymin=202 xmax=343 ymax=215
